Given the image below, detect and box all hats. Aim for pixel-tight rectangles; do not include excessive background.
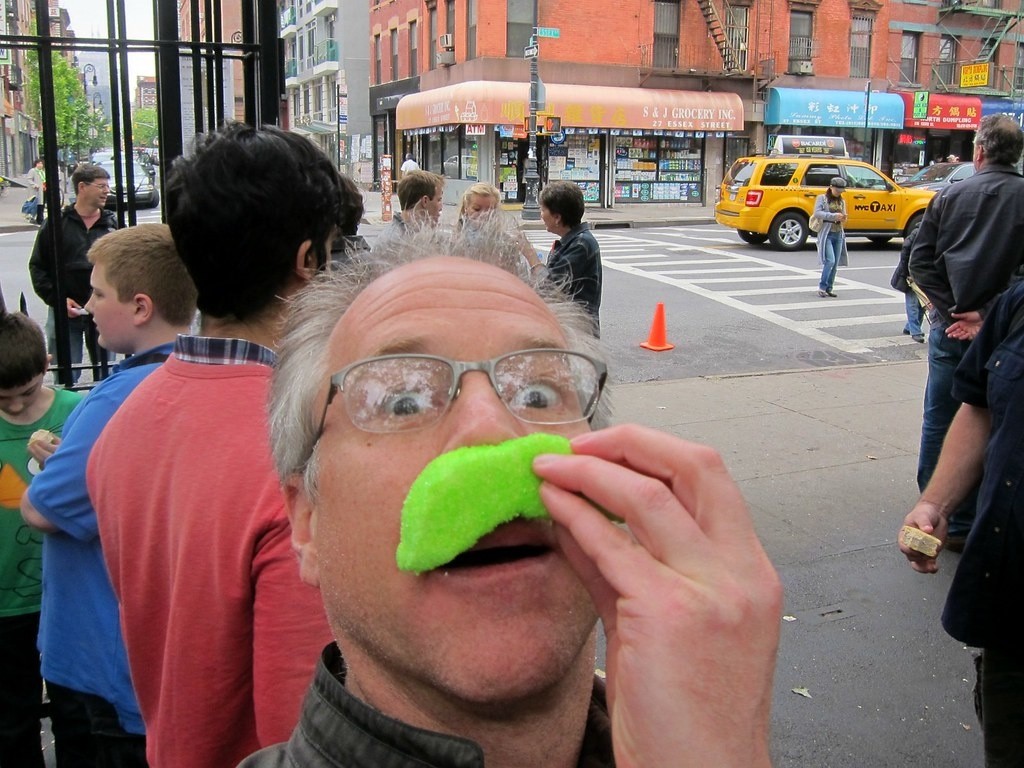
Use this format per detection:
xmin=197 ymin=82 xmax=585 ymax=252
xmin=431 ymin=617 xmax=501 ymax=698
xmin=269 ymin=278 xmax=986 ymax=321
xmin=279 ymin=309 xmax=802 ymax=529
xmin=830 ymin=177 xmax=847 ymax=192
xmin=947 ymin=155 xmax=955 ymax=159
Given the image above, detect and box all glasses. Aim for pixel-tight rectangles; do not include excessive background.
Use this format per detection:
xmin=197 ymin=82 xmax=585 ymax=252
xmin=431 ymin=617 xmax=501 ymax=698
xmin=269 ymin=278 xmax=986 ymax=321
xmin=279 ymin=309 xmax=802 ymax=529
xmin=86 ymin=182 xmax=109 ymax=189
xmin=311 ymin=348 xmax=608 ymax=455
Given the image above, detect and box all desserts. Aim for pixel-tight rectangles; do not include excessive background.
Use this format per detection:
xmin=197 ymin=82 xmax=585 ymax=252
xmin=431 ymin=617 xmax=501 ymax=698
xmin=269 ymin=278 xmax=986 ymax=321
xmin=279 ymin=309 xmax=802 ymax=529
xmin=27 ymin=430 xmax=54 ymax=444
xmin=901 ymin=526 xmax=942 ymax=557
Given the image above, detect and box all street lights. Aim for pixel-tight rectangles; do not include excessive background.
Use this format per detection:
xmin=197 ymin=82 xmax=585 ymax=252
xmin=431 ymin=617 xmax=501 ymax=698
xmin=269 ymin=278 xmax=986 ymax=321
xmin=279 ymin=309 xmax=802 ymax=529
xmin=92 ymin=92 xmax=103 ymax=137
xmin=84 ymin=63 xmax=98 ymax=95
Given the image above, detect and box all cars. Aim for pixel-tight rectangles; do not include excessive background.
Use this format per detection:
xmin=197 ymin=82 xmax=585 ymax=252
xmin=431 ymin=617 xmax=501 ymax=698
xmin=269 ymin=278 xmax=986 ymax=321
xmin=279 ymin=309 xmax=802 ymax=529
xmin=94 ymin=148 xmax=159 ymax=183
xmin=896 ymin=162 xmax=976 ymax=193
xmin=93 ymin=161 xmax=158 ymax=211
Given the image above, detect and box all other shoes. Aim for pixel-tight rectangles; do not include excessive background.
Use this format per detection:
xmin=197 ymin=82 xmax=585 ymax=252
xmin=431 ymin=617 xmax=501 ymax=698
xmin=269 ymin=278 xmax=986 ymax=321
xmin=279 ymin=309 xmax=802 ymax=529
xmin=903 ymin=329 xmax=925 ymax=343
xmin=817 ymin=289 xmax=838 ymax=298
xmin=945 ymin=531 xmax=969 ymax=552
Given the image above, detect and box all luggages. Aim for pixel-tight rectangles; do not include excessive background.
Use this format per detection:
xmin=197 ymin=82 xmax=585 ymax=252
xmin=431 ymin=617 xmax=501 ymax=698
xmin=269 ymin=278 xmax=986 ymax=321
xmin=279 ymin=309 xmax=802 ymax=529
xmin=22 ymin=187 xmax=39 ymax=223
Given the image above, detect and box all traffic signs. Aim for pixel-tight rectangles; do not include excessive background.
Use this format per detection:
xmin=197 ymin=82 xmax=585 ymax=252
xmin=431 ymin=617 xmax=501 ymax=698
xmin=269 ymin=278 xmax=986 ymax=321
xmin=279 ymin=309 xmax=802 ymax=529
xmin=523 ymin=44 xmax=539 ymax=60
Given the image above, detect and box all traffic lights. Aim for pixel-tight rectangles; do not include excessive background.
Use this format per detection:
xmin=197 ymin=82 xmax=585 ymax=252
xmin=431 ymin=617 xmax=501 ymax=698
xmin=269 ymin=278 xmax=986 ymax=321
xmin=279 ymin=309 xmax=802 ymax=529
xmin=524 ymin=116 xmax=536 ymax=133
xmin=545 ymin=116 xmax=561 ymax=134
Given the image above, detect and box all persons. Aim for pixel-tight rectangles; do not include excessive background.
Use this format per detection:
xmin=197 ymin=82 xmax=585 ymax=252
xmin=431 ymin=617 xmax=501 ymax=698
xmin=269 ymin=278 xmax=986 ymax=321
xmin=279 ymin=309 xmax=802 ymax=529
xmin=400 ymin=152 xmax=420 ymax=179
xmin=809 ymin=177 xmax=849 ymax=298
xmin=447 ymin=182 xmax=522 ymax=276
xmin=507 ymin=181 xmax=602 ymax=342
xmin=890 ymin=112 xmax=1024 ymax=768
xmin=27 ymin=164 xmax=127 ymax=395
xmin=369 ymin=170 xmax=446 ymax=279
xmin=21 ymin=158 xmax=49 ymax=227
xmin=230 ymin=205 xmax=780 ymax=768
xmin=0 ymin=119 xmax=377 ymax=768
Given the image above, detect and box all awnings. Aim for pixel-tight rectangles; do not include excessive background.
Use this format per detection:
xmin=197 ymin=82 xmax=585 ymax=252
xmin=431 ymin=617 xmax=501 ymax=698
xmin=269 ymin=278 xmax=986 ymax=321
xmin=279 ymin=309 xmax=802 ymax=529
xmin=767 ymin=87 xmax=1024 ymax=130
xmin=395 ymin=80 xmax=743 ymax=132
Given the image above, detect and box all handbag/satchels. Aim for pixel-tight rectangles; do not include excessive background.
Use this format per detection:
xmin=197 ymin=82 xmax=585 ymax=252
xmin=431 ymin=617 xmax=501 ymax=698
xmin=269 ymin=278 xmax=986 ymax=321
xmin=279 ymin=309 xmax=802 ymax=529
xmin=808 ymin=194 xmax=826 ymax=232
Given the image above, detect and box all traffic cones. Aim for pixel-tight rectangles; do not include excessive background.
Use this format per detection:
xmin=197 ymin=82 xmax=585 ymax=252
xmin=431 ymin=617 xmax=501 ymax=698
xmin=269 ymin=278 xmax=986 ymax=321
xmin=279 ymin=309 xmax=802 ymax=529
xmin=639 ymin=302 xmax=675 ymax=352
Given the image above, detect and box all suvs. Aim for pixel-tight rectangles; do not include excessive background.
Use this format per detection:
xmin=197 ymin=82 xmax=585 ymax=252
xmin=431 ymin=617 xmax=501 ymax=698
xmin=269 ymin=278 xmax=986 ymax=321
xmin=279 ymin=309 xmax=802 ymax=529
xmin=714 ymin=153 xmax=938 ymax=251
